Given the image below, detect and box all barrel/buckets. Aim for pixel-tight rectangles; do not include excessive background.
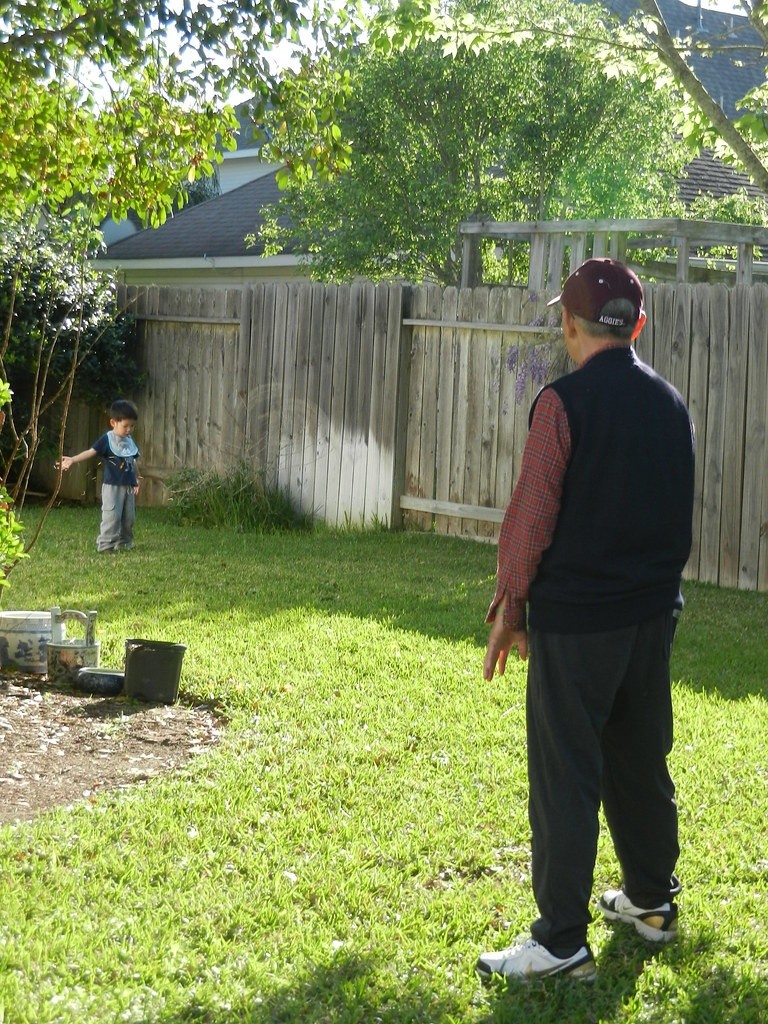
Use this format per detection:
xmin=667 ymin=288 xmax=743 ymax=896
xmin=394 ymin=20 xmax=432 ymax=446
xmin=124 ymin=638 xmax=188 ymax=704
xmin=45 ymin=605 xmax=101 ymax=685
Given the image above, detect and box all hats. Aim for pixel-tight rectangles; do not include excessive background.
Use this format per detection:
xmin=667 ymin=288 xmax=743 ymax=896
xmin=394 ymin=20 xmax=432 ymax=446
xmin=546 ymin=259 xmax=646 ymax=327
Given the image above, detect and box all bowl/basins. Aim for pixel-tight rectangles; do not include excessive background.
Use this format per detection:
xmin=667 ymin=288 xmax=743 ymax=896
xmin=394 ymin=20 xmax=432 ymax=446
xmin=77 ymin=666 xmax=125 ymax=695
xmin=1 ymin=611 xmax=65 ymax=674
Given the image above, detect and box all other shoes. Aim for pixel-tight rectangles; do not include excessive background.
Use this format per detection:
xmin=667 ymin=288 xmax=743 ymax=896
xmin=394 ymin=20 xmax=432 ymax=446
xmin=104 ymin=546 xmax=120 ymax=554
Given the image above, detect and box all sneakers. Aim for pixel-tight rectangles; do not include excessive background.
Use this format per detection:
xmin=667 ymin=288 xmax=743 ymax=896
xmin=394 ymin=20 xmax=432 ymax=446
xmin=600 ymin=885 xmax=679 ymax=943
xmin=476 ymin=934 xmax=599 ymax=985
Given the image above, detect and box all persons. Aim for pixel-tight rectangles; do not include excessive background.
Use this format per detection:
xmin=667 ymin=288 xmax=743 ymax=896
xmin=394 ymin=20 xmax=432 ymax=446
xmin=476 ymin=255 xmax=695 ymax=987
xmin=55 ymin=400 xmax=144 ymax=552
